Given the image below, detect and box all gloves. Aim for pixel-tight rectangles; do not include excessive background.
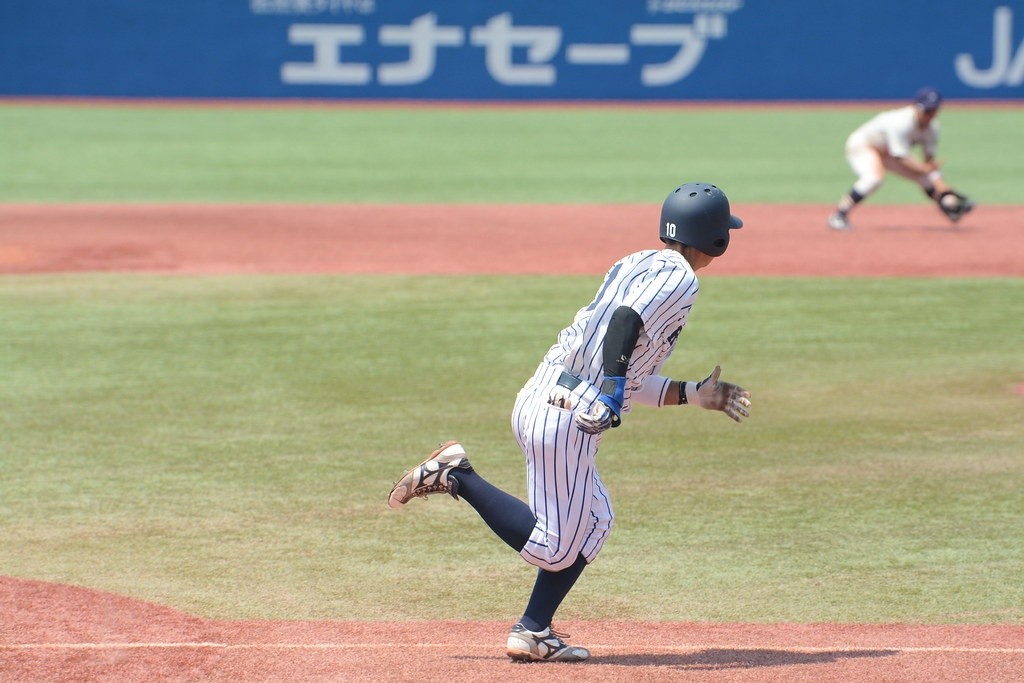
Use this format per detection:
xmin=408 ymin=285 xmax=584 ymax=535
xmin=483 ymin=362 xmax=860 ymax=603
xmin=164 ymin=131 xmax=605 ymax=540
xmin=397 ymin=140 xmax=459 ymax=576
xmin=574 ymin=401 xmax=614 ymax=435
xmin=686 ymin=366 xmax=752 ymax=423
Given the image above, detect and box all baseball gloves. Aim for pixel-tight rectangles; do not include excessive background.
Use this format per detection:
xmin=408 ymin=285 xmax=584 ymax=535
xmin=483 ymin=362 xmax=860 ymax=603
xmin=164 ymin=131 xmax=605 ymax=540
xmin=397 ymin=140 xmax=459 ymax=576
xmin=938 ymin=189 xmax=974 ymax=222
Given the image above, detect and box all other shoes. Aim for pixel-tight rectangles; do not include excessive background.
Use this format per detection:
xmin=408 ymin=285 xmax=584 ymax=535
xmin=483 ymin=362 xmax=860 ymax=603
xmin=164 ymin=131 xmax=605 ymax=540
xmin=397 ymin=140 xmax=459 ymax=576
xmin=827 ymin=214 xmax=847 ymax=230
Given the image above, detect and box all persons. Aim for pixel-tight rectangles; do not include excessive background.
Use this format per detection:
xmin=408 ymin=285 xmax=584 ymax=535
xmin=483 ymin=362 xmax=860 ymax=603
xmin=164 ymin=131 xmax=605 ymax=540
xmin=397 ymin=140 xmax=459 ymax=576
xmin=827 ymin=88 xmax=976 ymax=231
xmin=387 ymin=183 xmax=752 ymax=661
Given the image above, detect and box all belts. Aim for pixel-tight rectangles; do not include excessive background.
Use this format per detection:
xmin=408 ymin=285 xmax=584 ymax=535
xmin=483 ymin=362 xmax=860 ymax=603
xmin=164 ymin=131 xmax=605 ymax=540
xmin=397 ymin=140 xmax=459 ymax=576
xmin=558 ymin=371 xmax=582 ymax=391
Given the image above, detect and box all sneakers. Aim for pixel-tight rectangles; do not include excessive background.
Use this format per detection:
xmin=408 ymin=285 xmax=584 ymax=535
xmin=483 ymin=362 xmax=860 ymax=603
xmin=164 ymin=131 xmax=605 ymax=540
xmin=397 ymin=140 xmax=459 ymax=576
xmin=387 ymin=442 xmax=472 ymax=508
xmin=507 ymin=622 xmax=590 ymax=662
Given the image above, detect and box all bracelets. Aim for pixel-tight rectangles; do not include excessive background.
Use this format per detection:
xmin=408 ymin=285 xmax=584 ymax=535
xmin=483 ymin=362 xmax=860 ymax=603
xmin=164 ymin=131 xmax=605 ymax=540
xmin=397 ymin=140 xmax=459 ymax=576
xmin=678 ymin=380 xmax=688 ymax=405
xmin=597 ymin=377 xmax=627 ymax=427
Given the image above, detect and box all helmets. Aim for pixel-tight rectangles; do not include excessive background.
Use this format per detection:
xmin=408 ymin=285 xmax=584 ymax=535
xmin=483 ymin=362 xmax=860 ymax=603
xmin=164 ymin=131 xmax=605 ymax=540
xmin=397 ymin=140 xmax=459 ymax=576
xmin=660 ymin=182 xmax=744 ymax=257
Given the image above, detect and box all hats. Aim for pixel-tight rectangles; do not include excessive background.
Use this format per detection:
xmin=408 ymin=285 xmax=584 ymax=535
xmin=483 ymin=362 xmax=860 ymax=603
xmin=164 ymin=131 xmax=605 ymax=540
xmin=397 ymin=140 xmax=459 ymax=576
xmin=917 ymin=87 xmax=941 ymax=109
